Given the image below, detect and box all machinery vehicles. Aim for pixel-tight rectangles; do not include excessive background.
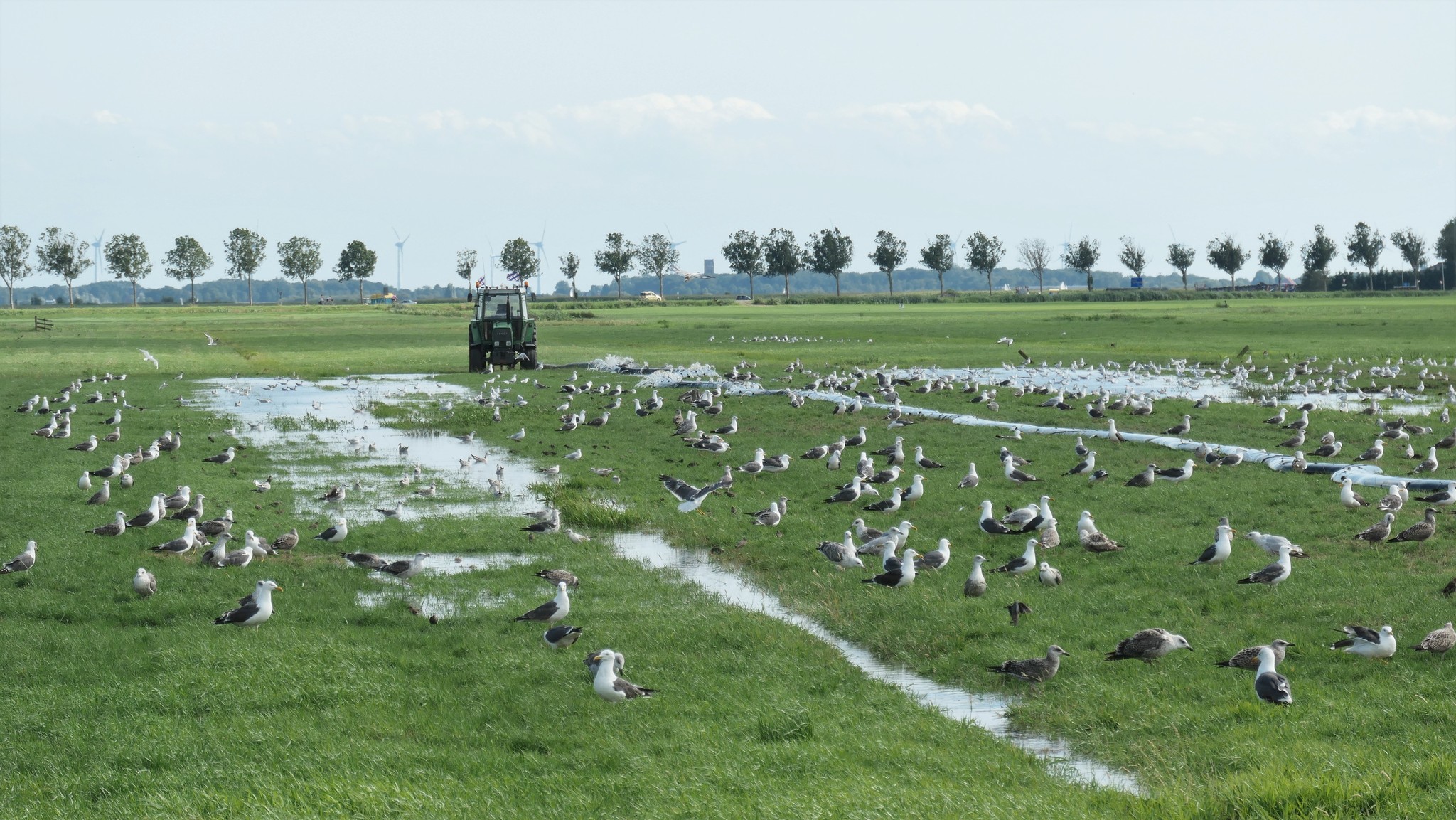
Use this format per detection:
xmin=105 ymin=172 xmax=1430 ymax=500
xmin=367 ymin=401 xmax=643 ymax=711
xmin=467 ymin=257 xmax=538 ymax=372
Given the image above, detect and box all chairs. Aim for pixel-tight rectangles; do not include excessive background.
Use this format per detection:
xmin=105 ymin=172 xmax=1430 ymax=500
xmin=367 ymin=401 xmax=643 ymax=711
xmin=496 ymin=304 xmax=507 ymax=317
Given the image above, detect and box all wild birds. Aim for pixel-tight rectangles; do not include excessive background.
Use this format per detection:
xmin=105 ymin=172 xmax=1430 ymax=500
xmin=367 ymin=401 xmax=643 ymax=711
xmin=0 ymin=330 xmax=1456 ymax=711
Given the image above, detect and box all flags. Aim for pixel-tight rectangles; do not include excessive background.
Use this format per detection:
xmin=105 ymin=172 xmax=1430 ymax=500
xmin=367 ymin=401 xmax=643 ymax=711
xmin=507 ymin=272 xmax=519 ymax=281
xmin=478 ymin=275 xmax=485 ymax=286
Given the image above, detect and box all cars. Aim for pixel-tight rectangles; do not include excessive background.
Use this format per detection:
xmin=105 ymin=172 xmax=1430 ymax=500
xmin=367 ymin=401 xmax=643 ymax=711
xmin=639 ymin=291 xmax=662 ymax=302
xmin=735 ymin=295 xmax=752 ymax=300
xmin=401 ymin=300 xmax=417 ymax=305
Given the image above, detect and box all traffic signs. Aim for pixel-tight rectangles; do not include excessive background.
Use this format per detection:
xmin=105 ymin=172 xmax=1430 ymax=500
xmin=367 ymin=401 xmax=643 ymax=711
xmin=1131 ymin=277 xmax=1143 ymax=288
